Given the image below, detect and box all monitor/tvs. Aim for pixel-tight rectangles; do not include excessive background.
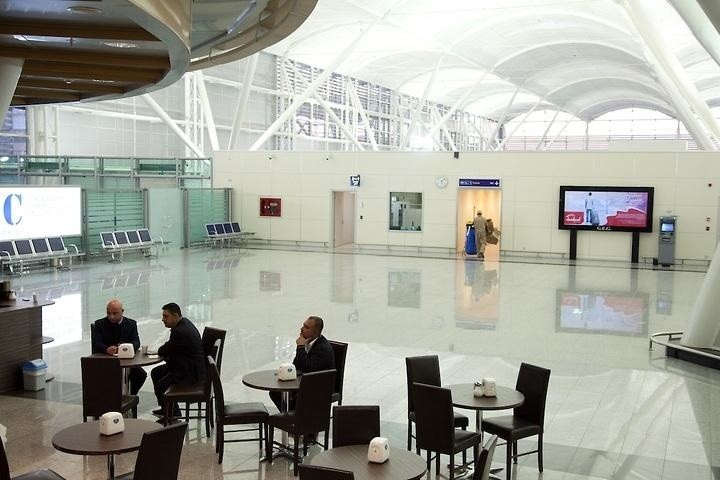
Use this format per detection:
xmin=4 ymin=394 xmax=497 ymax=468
xmin=660 ymin=222 xmax=674 ymax=233
xmin=558 ymin=186 xmax=654 ymax=233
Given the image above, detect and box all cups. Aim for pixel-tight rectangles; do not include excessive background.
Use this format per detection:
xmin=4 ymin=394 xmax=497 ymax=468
xmin=9 ymin=293 xmax=17 ymax=301
xmin=141 ymin=345 xmax=148 ymax=355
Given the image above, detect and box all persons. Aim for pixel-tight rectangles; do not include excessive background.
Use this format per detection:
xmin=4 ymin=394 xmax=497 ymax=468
xmin=149 ymin=302 xmax=207 ymax=424
xmin=269 ymin=316 xmax=338 ymax=442
xmin=91 ymin=299 xmax=148 ymax=397
xmin=473 ymin=210 xmax=491 ymax=258
xmin=585 ymin=192 xmax=594 ymax=223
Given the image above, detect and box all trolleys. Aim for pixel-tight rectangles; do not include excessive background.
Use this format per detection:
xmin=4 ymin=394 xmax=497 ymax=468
xmin=462 ymin=221 xmax=480 ymax=257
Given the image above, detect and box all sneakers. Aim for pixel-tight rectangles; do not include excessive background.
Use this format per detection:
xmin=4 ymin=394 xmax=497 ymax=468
xmin=479 ymin=252 xmax=484 ymax=258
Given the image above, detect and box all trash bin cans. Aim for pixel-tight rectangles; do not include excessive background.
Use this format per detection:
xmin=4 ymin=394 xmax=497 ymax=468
xmin=22 ymin=358 xmax=48 ymax=392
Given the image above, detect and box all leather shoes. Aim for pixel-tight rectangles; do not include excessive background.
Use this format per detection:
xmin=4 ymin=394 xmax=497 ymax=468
xmin=156 ymin=408 xmax=182 ymax=424
xmin=152 ymin=405 xmax=179 ymax=415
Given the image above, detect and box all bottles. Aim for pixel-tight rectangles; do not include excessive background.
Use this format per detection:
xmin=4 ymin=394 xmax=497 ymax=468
xmin=472 ymin=382 xmax=484 ymax=398
xmin=33 ymin=294 xmax=39 ymax=304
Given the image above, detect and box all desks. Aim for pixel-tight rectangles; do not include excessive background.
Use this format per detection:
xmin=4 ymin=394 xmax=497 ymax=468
xmin=241 ymin=370 xmax=309 ymax=463
xmin=51 ymin=419 xmax=164 ymax=480
xmin=84 ymin=349 xmax=164 ymax=419
xmin=311 ymin=447 xmax=429 ymax=480
xmin=440 ymin=384 xmax=526 ymax=478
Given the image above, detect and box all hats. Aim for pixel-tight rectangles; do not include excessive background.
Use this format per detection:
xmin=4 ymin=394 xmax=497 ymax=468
xmin=476 ymin=210 xmax=481 ymax=214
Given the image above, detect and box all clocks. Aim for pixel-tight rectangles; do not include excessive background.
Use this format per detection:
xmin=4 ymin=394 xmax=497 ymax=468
xmin=434 ymin=174 xmax=448 ymax=188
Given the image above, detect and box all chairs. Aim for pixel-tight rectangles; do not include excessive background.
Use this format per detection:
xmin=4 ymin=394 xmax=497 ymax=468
xmin=100 ymin=229 xmax=165 ymax=264
xmin=114 ymin=421 xmax=188 ymax=480
xmin=297 ymin=464 xmax=354 ymax=480
xmin=207 ymin=356 xmax=269 ymax=464
xmin=267 ymin=369 xmax=337 ymax=477
xmin=81 ymin=322 xmax=139 ymax=421
xmin=309 ymin=341 xmax=348 ymax=445
xmin=2 ymin=440 xmax=65 ymax=480
xmin=163 ymin=326 xmax=227 ymax=438
xmin=205 ymin=222 xmax=256 ymax=249
xmin=405 ymin=353 xmax=469 ymax=469
xmin=480 ymin=362 xmax=550 ymax=480
xmin=332 ymin=405 xmax=380 ymax=447
xmin=412 ymin=382 xmax=482 ymax=480
xmin=0 ymin=234 xmax=78 ymax=279
